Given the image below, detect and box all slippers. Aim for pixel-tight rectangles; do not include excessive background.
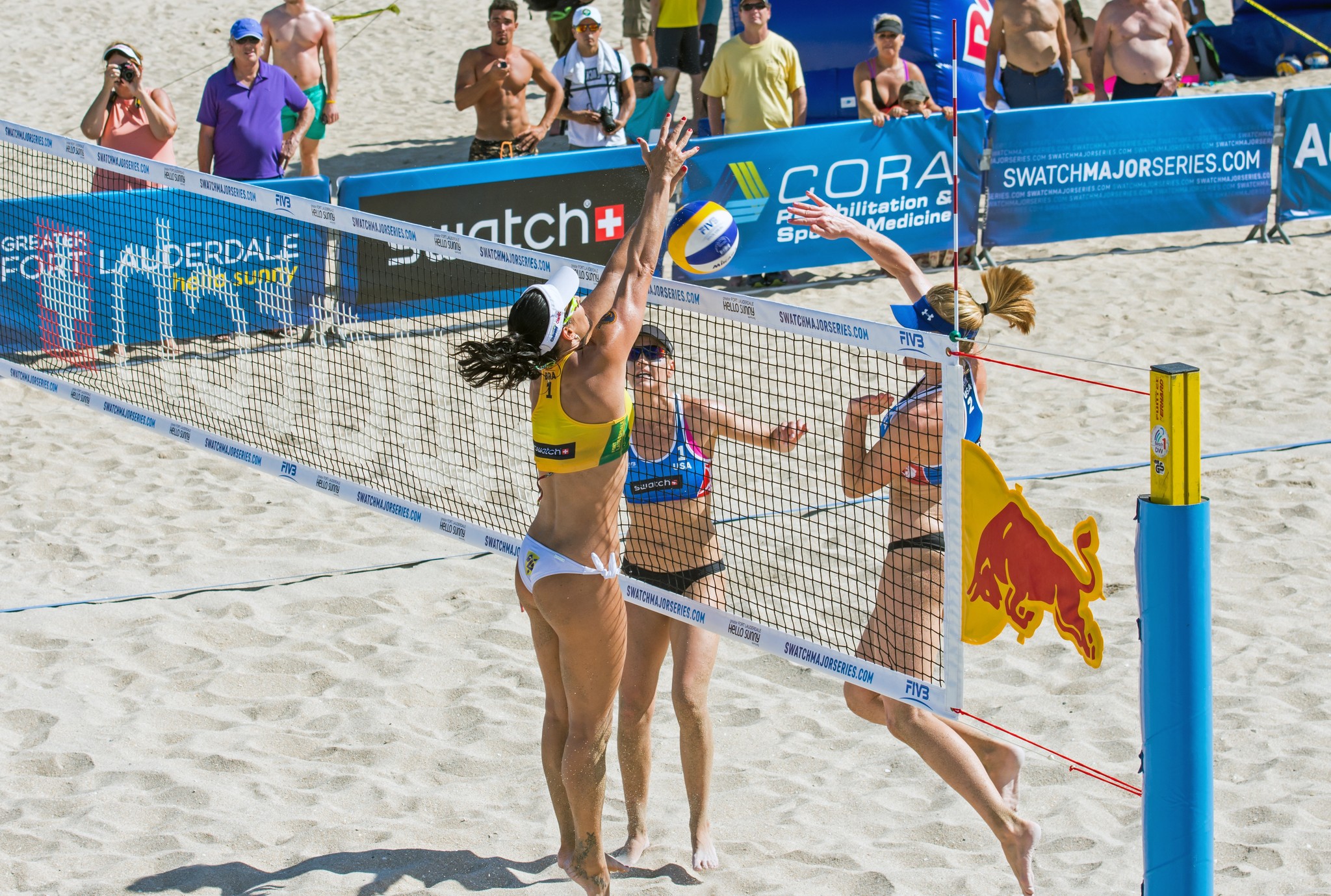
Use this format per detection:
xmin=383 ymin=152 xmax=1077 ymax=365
xmin=266 ymin=327 xmax=297 ymax=338
xmin=214 ymin=333 xmax=236 ymax=342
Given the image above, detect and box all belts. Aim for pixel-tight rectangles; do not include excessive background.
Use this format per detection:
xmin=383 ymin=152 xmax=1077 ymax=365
xmin=1006 ymin=61 xmax=1050 ymax=77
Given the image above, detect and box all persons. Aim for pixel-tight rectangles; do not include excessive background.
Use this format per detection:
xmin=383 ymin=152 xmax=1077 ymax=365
xmin=524 ymin=0 xmax=595 ymax=135
xmin=985 ymin=0 xmax=1074 ymax=109
xmin=451 ymin=112 xmax=700 ymax=896
xmin=652 ymin=0 xmax=706 ymax=138
xmin=623 ymin=0 xmax=652 ymax=69
xmin=81 ymin=40 xmax=179 ymax=192
xmin=624 ymin=63 xmax=680 ymax=146
xmin=647 ymin=0 xmax=665 ymax=68
xmin=258 ymin=0 xmax=340 ymax=176
xmin=607 ymin=325 xmax=809 ymax=874
xmin=1064 ymin=0 xmax=1200 ymax=102
xmin=785 ymin=192 xmax=1044 ymax=895
xmin=699 ymin=0 xmax=807 ymax=137
xmin=853 ymin=12 xmax=953 ymax=127
xmin=455 ymin=0 xmax=565 ymax=163
xmin=545 ymin=6 xmax=637 ymax=152
xmin=699 ymin=0 xmax=723 ymax=115
xmin=196 ymin=18 xmax=316 ymax=183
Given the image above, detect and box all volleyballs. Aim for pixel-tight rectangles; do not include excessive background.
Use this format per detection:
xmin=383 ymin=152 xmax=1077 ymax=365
xmin=666 ymin=200 xmax=739 ymax=275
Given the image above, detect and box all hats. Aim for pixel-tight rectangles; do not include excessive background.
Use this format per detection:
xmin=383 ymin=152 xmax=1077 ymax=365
xmin=889 ymin=294 xmax=979 ymax=341
xmin=230 ymin=18 xmax=263 ymax=41
xmin=739 ymin=0 xmax=772 ymax=9
xmin=874 ymin=18 xmax=902 ymax=35
xmin=631 ymin=63 xmax=652 ymax=77
xmin=521 ymin=266 xmax=580 ymax=357
xmin=640 ymin=324 xmax=674 ymax=356
xmin=898 ymin=80 xmax=929 ymax=103
xmin=572 ymin=6 xmax=602 ymax=26
xmin=104 ymin=43 xmax=142 ymax=67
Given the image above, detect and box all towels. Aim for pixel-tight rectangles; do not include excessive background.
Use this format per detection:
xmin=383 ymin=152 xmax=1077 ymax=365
xmin=562 ymin=34 xmax=621 ymax=85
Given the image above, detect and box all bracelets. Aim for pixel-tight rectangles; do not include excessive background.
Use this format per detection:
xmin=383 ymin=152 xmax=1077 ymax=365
xmin=325 ymin=100 xmax=337 ymax=104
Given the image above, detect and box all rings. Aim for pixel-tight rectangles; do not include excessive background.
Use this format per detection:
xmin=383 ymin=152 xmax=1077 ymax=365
xmin=110 ymin=74 xmax=114 ymax=78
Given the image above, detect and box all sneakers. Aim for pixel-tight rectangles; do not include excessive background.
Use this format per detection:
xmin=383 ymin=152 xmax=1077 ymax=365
xmin=764 ymin=271 xmax=786 ymax=287
xmin=746 ymin=273 xmax=766 ymax=289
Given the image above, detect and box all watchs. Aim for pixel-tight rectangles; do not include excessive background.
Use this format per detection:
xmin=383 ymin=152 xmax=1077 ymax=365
xmin=1174 ymin=73 xmax=1182 ymax=81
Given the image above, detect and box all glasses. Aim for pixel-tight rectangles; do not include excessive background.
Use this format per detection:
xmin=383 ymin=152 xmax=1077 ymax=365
xmin=740 ymin=2 xmax=765 ymax=11
xmin=633 ymin=75 xmax=651 ymax=83
xmin=563 ymin=296 xmax=579 ymax=326
xmin=235 ymin=37 xmax=260 ymax=44
xmin=573 ymin=23 xmax=600 ymax=33
xmin=627 ymin=344 xmax=674 ymax=362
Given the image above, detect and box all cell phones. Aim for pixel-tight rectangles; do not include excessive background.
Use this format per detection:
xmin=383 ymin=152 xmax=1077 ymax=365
xmin=500 ymin=62 xmax=507 ymax=68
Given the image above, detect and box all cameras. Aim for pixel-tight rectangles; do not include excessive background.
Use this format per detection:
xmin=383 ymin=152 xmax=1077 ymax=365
xmin=114 ymin=62 xmax=134 ymax=83
xmin=595 ymin=106 xmax=616 ymax=133
